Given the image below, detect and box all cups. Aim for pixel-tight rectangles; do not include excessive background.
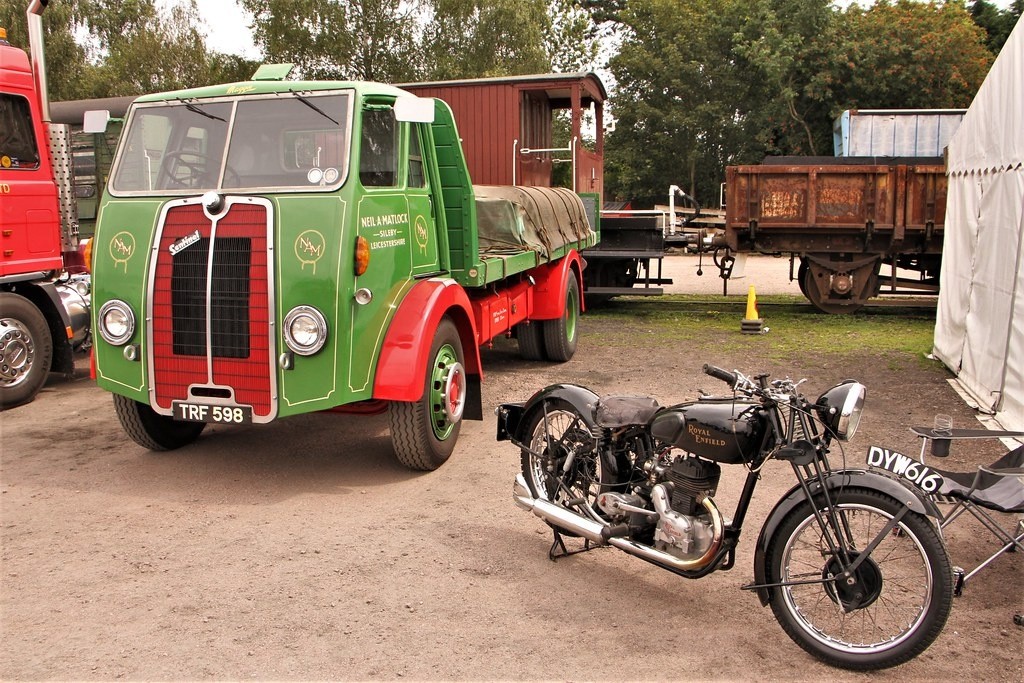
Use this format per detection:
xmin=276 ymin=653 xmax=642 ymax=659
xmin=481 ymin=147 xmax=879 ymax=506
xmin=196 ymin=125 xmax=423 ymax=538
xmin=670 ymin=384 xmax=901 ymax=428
xmin=931 ymin=414 xmax=952 ymax=458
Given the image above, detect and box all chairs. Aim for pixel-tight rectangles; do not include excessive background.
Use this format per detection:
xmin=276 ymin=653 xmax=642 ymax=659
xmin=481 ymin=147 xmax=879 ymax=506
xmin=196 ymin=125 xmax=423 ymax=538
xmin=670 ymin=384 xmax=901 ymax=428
xmin=892 ymin=427 xmax=1024 ymax=598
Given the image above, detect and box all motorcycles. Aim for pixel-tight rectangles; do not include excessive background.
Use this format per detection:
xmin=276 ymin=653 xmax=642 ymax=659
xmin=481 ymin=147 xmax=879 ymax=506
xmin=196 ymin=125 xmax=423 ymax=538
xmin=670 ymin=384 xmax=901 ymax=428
xmin=496 ymin=363 xmax=955 ymax=672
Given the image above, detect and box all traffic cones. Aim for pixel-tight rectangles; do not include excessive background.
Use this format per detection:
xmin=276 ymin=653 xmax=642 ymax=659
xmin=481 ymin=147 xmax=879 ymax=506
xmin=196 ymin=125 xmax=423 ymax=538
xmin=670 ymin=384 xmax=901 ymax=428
xmin=740 ymin=284 xmax=764 ymax=335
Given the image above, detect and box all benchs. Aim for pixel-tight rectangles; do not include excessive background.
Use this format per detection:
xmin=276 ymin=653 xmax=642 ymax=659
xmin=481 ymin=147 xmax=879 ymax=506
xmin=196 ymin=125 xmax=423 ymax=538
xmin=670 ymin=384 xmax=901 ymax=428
xmin=235 ymin=170 xmax=396 ymax=188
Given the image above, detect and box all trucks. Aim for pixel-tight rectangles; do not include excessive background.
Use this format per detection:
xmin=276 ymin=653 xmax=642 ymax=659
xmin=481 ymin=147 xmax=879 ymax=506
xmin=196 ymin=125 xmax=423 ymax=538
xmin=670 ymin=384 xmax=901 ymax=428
xmin=89 ymin=61 xmax=601 ymax=474
xmin=0 ymin=29 xmax=206 ymax=413
